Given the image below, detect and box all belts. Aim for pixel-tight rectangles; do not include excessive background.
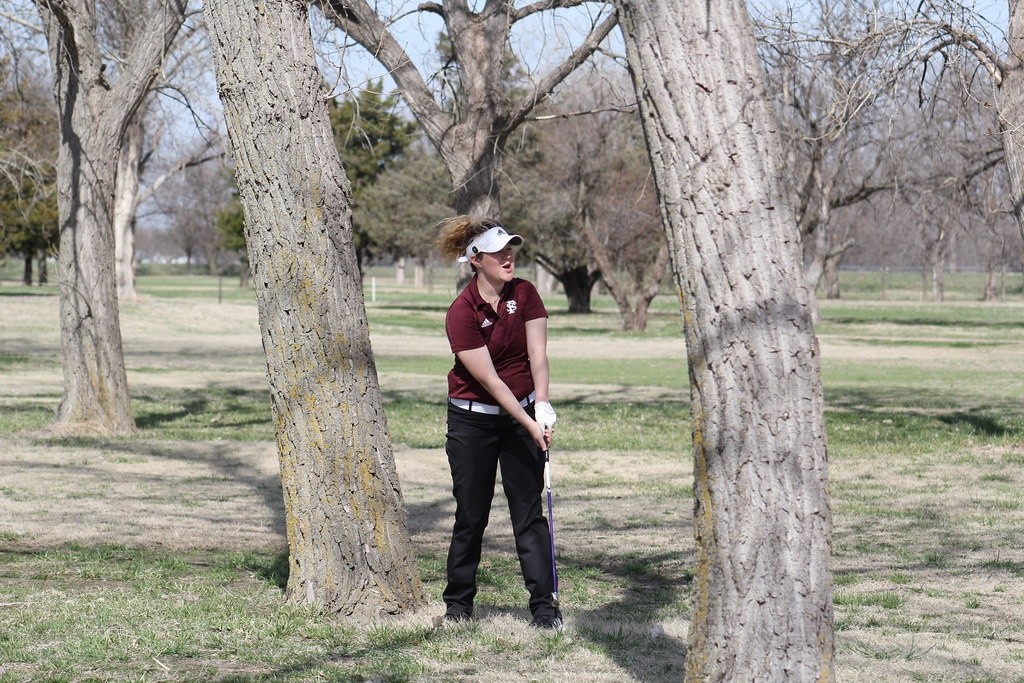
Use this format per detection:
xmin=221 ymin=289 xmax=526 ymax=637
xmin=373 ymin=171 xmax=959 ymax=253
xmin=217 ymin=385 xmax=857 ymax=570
xmin=448 ymin=391 xmax=536 ymax=415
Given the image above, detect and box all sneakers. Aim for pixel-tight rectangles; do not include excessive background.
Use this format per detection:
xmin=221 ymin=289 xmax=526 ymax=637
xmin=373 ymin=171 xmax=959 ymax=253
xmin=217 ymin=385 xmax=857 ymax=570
xmin=528 ymin=616 xmax=563 ymax=631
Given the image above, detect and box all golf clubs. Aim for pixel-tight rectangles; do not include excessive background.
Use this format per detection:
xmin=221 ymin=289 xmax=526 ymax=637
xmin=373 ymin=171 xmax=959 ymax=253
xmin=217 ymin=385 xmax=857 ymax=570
xmin=543 ymin=425 xmax=563 ymax=634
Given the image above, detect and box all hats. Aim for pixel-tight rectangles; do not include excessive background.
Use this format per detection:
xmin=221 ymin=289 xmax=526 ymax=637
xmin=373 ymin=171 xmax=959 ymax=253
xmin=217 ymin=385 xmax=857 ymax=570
xmin=458 ymin=227 xmax=524 ymax=262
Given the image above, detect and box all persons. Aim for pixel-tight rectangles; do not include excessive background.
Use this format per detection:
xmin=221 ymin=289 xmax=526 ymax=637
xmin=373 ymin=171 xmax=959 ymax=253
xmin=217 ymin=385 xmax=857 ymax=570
xmin=427 ymin=215 xmax=565 ymax=629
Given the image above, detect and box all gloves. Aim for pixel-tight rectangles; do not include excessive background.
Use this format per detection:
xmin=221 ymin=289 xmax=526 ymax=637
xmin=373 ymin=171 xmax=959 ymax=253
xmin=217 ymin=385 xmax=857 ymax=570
xmin=534 ymin=401 xmax=557 ymax=436
